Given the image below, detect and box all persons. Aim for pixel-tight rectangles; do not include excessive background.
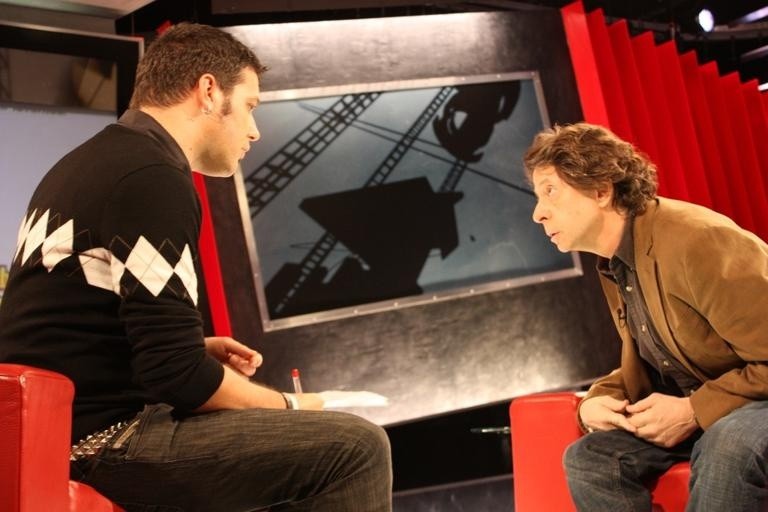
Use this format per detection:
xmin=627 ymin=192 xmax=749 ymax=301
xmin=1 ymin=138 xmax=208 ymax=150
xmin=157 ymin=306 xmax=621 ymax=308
xmin=1 ymin=24 xmax=395 ymax=511
xmin=522 ymin=121 xmax=768 ymax=512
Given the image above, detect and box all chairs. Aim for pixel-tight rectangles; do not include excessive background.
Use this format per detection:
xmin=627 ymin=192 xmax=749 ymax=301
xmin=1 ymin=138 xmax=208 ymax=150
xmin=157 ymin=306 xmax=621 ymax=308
xmin=1 ymin=362 xmax=124 ymax=512
xmin=507 ymin=390 xmax=694 ymax=512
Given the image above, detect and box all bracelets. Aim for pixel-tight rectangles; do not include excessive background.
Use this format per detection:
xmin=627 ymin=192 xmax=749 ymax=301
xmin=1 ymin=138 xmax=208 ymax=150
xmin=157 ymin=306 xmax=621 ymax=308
xmin=283 ymin=389 xmax=299 ymax=412
